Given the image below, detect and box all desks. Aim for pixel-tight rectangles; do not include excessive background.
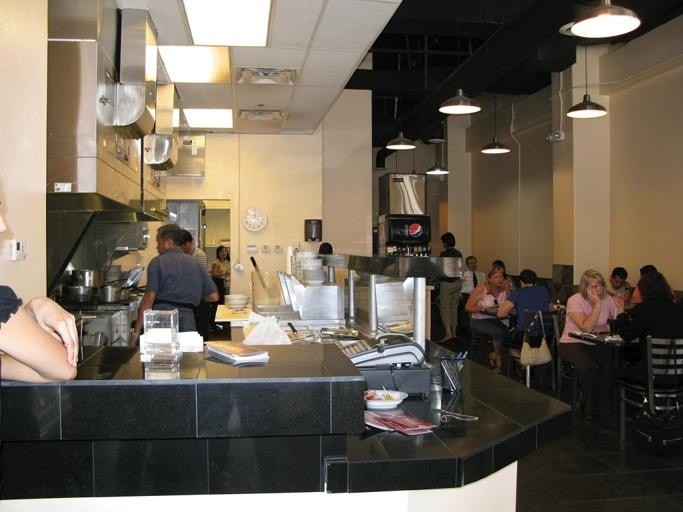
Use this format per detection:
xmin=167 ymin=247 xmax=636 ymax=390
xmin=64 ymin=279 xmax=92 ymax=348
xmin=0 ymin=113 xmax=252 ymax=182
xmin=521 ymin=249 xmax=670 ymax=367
xmin=568 ymin=331 xmax=622 ymax=347
xmin=479 ymin=309 xmax=515 ymax=377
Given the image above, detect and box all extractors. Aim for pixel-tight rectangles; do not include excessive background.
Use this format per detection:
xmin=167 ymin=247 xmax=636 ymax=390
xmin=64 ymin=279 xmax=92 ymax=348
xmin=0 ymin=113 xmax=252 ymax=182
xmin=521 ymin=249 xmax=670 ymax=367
xmin=46 ymin=2 xmax=155 ymax=294
xmin=94 ymin=81 xmax=179 ymax=251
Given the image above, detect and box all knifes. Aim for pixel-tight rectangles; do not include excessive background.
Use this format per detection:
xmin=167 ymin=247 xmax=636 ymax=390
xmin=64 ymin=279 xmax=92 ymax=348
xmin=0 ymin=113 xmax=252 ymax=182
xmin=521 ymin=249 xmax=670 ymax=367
xmin=250 ymin=256 xmax=266 ymax=289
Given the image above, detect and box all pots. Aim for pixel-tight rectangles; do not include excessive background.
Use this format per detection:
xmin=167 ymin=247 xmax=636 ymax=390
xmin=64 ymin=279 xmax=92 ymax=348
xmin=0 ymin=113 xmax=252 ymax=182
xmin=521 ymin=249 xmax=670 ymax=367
xmin=70 ymin=269 xmax=104 ymax=289
xmin=68 ymin=285 xmax=91 ymax=296
xmin=98 ymin=285 xmax=138 ymax=303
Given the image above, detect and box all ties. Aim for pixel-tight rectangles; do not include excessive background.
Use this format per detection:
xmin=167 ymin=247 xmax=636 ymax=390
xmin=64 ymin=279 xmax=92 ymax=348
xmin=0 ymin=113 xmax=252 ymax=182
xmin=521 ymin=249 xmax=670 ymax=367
xmin=473 ymin=272 xmax=477 ymax=287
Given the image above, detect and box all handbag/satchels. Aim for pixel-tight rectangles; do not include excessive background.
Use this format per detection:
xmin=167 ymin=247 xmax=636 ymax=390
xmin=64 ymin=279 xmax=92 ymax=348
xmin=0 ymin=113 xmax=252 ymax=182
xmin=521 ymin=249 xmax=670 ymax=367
xmin=520 ymin=336 xmax=552 ymax=366
xmin=631 ymin=405 xmax=683 ymax=457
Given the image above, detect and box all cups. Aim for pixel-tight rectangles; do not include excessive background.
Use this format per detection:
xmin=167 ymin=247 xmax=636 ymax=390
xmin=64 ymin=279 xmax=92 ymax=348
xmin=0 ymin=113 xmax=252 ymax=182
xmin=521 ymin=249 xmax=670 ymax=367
xmin=285 ymin=246 xmax=295 ymax=275
xmin=439 ymin=358 xmax=465 ymax=394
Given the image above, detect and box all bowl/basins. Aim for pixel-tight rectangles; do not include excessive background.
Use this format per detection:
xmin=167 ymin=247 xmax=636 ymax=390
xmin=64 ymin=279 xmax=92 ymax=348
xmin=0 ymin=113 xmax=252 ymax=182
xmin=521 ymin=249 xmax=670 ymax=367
xmin=223 ymin=294 xmax=249 ymax=308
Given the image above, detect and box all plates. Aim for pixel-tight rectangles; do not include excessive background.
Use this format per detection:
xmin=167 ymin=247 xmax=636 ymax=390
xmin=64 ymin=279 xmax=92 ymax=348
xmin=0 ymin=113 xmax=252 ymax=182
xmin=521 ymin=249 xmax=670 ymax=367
xmin=486 ymin=308 xmax=500 ymax=312
xmin=364 ymin=389 xmax=409 ymax=409
xmin=290 ymin=251 xmax=325 ymax=284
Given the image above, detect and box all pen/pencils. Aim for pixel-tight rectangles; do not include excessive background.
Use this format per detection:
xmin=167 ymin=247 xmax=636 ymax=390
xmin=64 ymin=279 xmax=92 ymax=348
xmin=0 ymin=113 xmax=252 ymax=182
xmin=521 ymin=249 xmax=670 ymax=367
xmin=440 ymin=351 xmax=468 ymax=389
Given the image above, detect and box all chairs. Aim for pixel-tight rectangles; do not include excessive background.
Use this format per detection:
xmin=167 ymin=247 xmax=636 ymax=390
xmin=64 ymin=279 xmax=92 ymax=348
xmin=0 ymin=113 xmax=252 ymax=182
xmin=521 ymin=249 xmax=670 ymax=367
xmin=507 ymin=310 xmax=556 ymax=391
xmin=553 ymin=314 xmax=583 ymax=425
xmin=616 ymin=335 xmax=683 ymax=441
xmin=469 ymin=313 xmax=491 ymax=364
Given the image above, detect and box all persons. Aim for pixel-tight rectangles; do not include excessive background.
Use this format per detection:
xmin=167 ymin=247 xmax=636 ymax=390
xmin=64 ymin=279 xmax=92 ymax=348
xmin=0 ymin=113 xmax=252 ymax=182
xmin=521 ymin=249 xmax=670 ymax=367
xmin=318 ymin=243 xmax=333 ymax=254
xmin=212 ymin=246 xmax=230 ymax=321
xmin=558 ymin=269 xmax=617 ymax=417
xmin=130 ymin=224 xmax=220 ymax=346
xmin=437 ymin=232 xmax=463 ymax=343
xmin=604 ymin=265 xmax=658 ymax=307
xmin=0 ymin=201 xmax=79 ymax=383
xmin=497 ymin=269 xmax=553 ymax=377
xmin=461 ymin=255 xmax=517 ymax=374
xmin=613 ymin=272 xmax=682 ymax=428
xmin=178 ymin=229 xmax=223 ymax=341
